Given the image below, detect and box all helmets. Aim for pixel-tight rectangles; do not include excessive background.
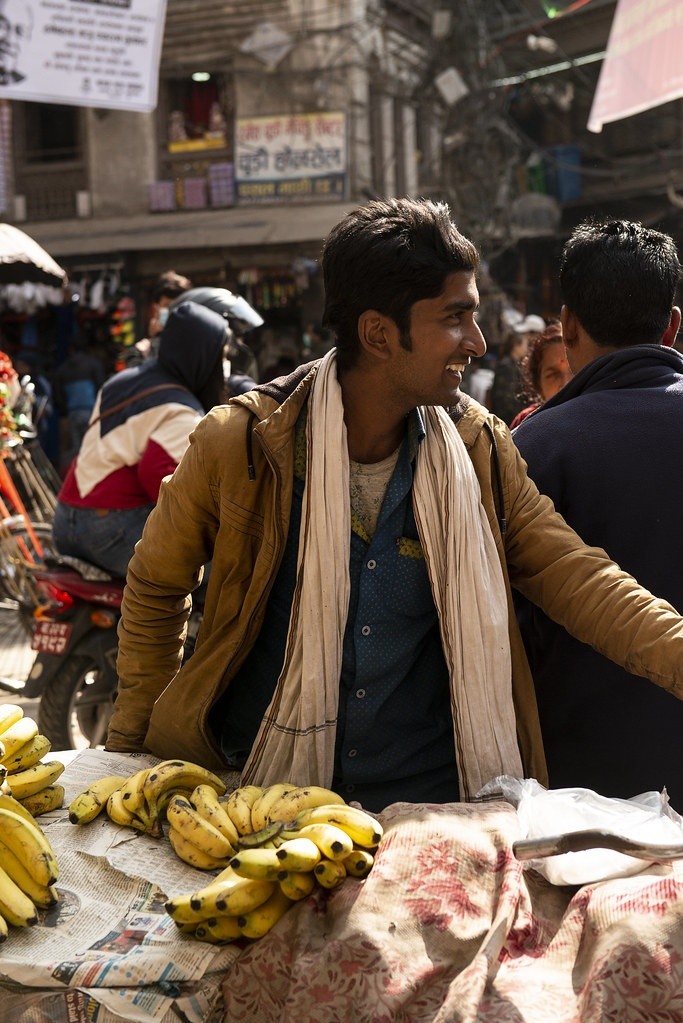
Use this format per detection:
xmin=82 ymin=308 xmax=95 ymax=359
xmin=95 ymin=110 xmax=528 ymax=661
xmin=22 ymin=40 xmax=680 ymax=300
xmin=172 ymin=286 xmax=264 ymax=336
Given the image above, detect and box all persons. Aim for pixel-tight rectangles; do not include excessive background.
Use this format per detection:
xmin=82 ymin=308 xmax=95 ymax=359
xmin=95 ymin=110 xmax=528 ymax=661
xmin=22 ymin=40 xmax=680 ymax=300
xmin=511 ymin=220 xmax=683 ymax=813
xmin=105 ymin=198 xmax=683 ymax=814
xmin=51 ymin=298 xmax=233 ymax=614
xmin=459 ymin=307 xmax=577 ymax=430
xmin=14 ymin=271 xmax=333 ymax=471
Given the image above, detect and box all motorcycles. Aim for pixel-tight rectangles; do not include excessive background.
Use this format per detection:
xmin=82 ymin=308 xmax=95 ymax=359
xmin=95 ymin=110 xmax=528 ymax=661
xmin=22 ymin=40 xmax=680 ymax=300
xmin=15 ymin=550 xmax=212 ymax=751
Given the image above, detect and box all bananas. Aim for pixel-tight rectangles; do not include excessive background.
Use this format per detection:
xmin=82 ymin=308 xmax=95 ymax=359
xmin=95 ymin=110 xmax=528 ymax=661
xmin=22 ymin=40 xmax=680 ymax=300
xmin=71 ymin=758 xmax=384 ymax=941
xmin=0 ymin=704 xmax=66 ymax=941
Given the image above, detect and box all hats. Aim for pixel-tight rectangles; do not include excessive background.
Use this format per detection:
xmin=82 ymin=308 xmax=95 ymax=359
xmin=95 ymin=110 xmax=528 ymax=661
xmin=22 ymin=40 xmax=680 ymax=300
xmin=514 ymin=315 xmax=546 ymax=333
